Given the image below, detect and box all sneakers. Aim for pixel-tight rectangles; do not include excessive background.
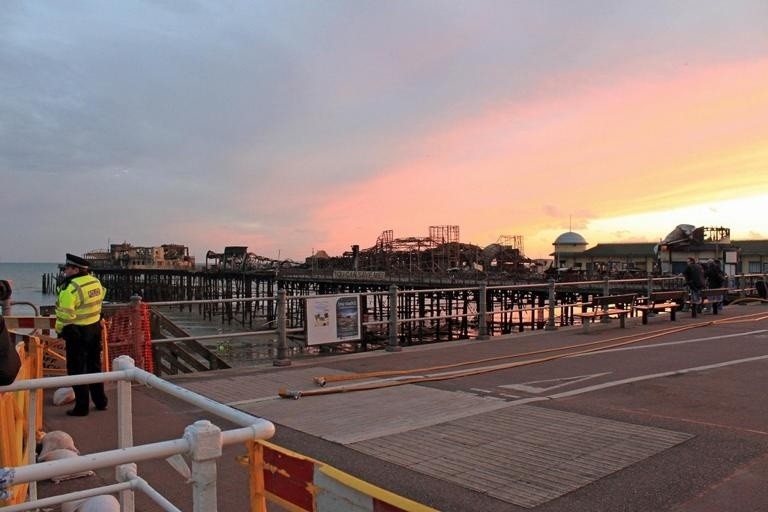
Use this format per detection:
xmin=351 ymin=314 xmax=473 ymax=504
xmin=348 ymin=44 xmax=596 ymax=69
xmin=66 ymin=404 xmax=109 ymax=416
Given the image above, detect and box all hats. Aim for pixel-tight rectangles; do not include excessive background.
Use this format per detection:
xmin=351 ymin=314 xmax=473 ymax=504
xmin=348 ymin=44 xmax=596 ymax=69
xmin=59 ymin=253 xmax=90 ymax=269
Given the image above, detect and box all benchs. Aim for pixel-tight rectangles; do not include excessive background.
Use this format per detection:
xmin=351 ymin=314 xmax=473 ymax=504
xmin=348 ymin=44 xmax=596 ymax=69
xmin=572 ymin=288 xmax=728 ymax=334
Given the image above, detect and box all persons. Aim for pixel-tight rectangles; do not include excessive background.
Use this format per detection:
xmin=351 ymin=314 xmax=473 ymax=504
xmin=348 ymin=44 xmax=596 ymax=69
xmin=0 ymin=278 xmax=22 ymax=386
xmin=678 ymin=257 xmax=706 ymax=313
xmin=653 ymin=262 xmax=660 ymax=275
xmin=595 ymin=257 xmax=619 ymax=273
xmin=704 ymin=258 xmax=728 ymax=312
xmin=54 ymin=253 xmax=109 ymax=416
